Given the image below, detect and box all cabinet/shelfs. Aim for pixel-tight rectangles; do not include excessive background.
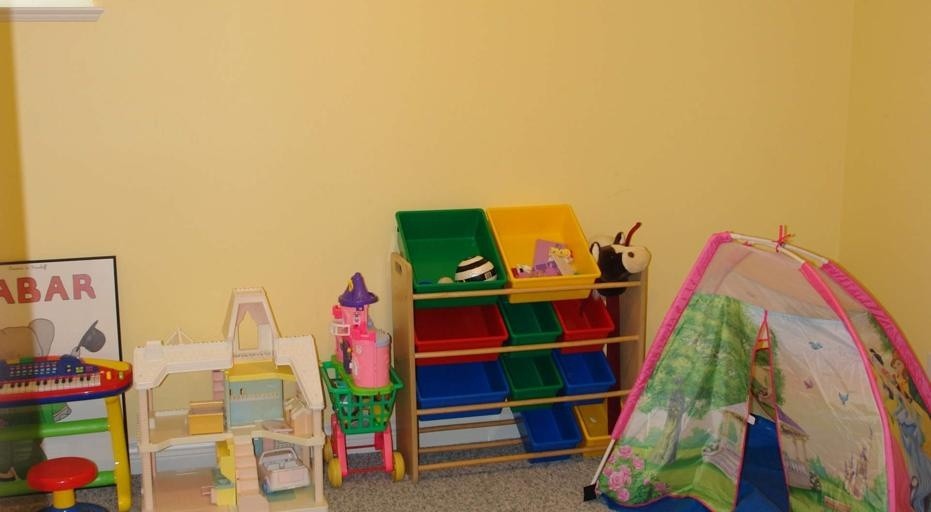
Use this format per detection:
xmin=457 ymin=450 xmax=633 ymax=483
xmin=387 ymin=238 xmax=648 ymax=484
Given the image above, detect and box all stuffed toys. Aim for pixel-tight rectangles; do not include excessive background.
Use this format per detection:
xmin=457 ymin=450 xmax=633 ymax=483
xmin=588 ymin=220 xmax=652 ymax=298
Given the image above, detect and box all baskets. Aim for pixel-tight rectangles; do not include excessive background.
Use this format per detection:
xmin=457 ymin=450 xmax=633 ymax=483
xmin=320 ymin=359 xmax=404 ymax=435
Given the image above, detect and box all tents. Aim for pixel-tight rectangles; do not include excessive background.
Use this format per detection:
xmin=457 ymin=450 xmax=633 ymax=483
xmin=584 ymin=224 xmax=930 ymax=510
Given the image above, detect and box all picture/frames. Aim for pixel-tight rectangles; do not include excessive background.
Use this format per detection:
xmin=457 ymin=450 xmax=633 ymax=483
xmin=0 ymin=256 xmax=126 ymax=481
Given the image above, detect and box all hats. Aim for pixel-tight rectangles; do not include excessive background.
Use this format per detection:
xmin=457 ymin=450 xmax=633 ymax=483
xmin=74 ymin=319 xmax=107 ymax=353
